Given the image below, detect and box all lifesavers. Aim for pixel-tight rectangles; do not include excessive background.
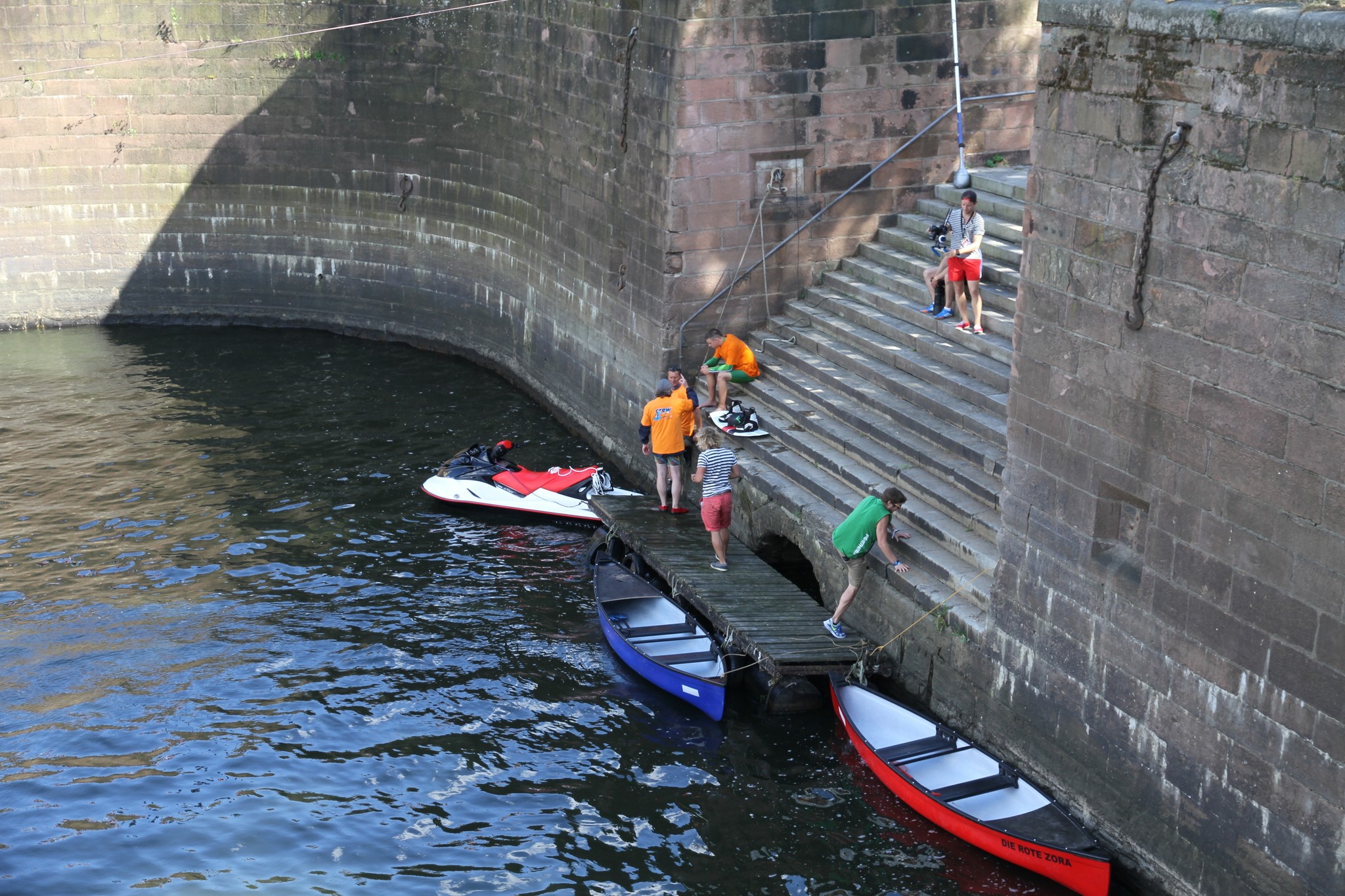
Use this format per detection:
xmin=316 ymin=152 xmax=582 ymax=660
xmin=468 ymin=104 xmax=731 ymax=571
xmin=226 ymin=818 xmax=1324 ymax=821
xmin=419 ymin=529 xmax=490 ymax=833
xmin=586 ymin=534 xmax=744 ymax=690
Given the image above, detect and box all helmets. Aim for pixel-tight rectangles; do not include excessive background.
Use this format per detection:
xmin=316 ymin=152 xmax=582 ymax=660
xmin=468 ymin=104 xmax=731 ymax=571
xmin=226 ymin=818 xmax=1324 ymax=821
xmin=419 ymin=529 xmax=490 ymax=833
xmin=727 ymin=413 xmax=746 ymax=427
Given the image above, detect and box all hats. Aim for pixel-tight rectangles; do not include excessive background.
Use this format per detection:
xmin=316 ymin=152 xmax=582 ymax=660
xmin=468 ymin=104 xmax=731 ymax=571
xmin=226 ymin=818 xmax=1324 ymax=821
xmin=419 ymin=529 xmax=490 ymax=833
xmin=658 ymin=379 xmax=672 ymax=391
xmin=962 ymin=190 xmax=976 ymax=202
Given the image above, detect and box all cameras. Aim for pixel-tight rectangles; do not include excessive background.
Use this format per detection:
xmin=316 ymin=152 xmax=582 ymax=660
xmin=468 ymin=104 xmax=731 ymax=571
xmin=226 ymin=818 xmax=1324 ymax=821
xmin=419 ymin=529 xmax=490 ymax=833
xmin=928 ymin=225 xmax=948 ymax=240
xmin=930 ymin=279 xmax=946 ymax=316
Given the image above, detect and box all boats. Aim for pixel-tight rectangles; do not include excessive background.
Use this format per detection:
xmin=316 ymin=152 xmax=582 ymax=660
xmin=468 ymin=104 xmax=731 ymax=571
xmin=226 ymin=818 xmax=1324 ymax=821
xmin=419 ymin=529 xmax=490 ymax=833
xmin=827 ymin=668 xmax=1112 ymax=896
xmin=592 ymin=549 xmax=730 ymax=723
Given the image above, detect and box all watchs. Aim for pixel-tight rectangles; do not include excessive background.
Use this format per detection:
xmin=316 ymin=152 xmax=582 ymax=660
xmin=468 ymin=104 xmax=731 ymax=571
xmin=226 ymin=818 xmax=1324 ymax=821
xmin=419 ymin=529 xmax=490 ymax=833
xmin=893 ymin=560 xmax=899 ymax=566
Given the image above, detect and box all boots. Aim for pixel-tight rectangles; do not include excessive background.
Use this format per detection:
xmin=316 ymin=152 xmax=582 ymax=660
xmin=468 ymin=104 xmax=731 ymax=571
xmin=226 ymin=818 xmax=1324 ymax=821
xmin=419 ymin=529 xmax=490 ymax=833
xmin=736 ymin=407 xmax=758 ymax=432
xmin=719 ymin=400 xmax=742 ymax=422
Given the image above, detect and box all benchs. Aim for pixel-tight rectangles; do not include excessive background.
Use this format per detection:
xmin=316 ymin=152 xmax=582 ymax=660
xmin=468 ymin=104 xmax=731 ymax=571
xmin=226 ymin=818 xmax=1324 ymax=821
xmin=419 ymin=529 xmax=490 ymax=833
xmin=618 ymin=623 xmax=695 ymax=639
xmin=646 ymin=652 xmax=720 ymax=663
xmin=874 ymin=734 xmax=956 ymax=762
xmin=930 ymin=774 xmax=1016 ymax=801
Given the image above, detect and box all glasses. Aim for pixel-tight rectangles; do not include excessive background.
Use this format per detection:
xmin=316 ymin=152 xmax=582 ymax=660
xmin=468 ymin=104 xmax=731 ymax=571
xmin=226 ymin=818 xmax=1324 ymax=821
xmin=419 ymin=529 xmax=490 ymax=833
xmin=894 ymin=504 xmax=901 ymax=510
xmin=669 ymin=367 xmax=681 ymax=370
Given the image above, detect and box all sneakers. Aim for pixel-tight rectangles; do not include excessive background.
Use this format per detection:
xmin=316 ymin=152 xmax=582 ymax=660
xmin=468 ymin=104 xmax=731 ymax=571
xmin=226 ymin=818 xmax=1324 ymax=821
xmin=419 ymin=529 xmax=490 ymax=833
xmin=671 ymin=507 xmax=688 ymax=514
xmin=973 ymin=324 xmax=983 ymax=333
xmin=711 ymin=561 xmax=728 ymax=571
xmin=683 ymin=445 xmax=692 ymax=467
xmin=659 ymin=505 xmax=669 ymax=512
xmin=823 ymin=618 xmax=846 ymax=638
xmin=666 ymin=491 xmax=672 ymax=498
xmin=716 ymin=553 xmax=729 ymax=563
xmin=920 ymin=302 xmax=934 ymax=313
xmin=955 ymin=320 xmax=970 ymax=330
xmin=934 ymin=308 xmax=954 ymax=319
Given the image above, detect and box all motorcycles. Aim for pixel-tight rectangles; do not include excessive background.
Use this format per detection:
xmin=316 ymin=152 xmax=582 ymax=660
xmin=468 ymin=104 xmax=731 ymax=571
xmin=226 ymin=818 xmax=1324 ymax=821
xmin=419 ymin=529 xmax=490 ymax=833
xmin=420 ymin=439 xmax=648 ymax=526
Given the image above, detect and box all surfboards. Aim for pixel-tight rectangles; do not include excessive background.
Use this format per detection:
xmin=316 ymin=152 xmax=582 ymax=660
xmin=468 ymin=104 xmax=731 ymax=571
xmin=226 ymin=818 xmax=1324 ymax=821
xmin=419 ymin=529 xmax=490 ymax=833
xmin=709 ymin=409 xmax=770 ymax=438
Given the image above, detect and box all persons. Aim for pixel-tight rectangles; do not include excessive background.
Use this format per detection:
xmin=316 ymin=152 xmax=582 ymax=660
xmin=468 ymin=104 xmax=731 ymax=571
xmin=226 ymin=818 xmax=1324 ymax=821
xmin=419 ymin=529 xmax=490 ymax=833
xmin=666 ymin=366 xmax=703 ymax=499
xmin=823 ymin=487 xmax=911 ymax=639
xmin=691 ymin=426 xmax=741 ymax=572
xmin=638 ymin=373 xmax=699 ymax=515
xmin=920 ymin=190 xmax=984 ymax=334
xmin=698 ymin=329 xmax=761 ymax=418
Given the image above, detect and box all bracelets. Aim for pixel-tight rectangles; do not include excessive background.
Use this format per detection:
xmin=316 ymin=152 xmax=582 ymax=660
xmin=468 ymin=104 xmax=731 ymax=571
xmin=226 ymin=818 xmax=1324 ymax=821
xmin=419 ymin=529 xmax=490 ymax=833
xmin=898 ymin=561 xmax=901 ymax=564
xmin=692 ymin=475 xmax=695 ymax=480
xmin=956 ymin=249 xmax=960 ymax=255
xmin=892 ymin=529 xmax=899 ymax=539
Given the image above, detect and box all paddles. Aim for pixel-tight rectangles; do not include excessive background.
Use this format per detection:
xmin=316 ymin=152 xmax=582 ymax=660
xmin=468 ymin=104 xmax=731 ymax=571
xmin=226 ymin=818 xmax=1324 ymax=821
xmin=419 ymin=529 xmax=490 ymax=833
xmin=609 ymin=613 xmax=635 ymax=634
